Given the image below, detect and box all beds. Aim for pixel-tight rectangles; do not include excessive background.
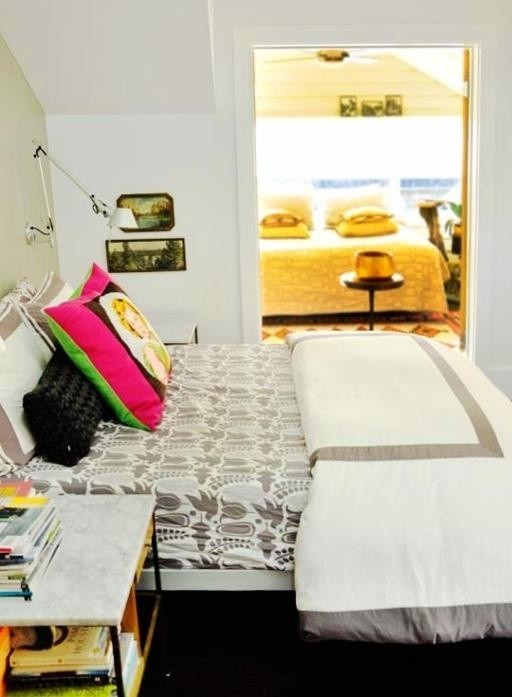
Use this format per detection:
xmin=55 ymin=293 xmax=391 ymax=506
xmin=259 ymin=209 xmax=441 ymax=321
xmin=0 ymin=340 xmax=509 ymax=595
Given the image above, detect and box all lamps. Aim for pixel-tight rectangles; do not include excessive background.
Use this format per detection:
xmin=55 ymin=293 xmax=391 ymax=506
xmin=25 ymin=138 xmax=140 ymax=249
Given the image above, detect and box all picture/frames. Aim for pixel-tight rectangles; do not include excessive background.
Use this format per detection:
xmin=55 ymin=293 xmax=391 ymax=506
xmin=115 ymin=193 xmax=175 ymax=233
xmin=104 ymin=237 xmax=188 ymax=273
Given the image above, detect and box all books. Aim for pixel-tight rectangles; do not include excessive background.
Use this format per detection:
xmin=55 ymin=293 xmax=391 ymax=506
xmin=0 ymin=481 xmax=139 ymax=697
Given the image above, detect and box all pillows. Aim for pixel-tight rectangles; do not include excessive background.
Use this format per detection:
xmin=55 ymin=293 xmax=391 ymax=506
xmin=0 ymin=261 xmax=173 ymax=477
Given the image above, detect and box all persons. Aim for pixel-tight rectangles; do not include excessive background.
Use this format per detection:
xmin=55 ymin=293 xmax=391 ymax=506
xmin=113 ymin=297 xmax=171 ymax=384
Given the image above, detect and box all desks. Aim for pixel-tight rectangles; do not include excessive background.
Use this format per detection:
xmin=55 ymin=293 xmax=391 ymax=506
xmin=338 ymin=271 xmax=405 ymax=331
xmin=0 ymin=493 xmax=163 ymax=697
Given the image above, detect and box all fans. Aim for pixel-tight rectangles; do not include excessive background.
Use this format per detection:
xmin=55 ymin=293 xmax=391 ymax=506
xmin=266 ymin=47 xmax=388 ymax=66
